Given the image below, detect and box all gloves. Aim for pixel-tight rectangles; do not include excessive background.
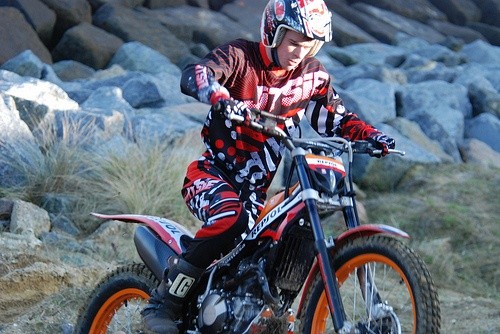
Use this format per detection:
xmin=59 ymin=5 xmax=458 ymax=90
xmin=215 ymin=98 xmax=253 ymax=131
xmin=367 ymin=131 xmax=396 ymax=154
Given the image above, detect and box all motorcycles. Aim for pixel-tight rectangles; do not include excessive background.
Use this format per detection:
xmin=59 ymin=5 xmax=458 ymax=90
xmin=74 ymin=107 xmax=442 ymax=334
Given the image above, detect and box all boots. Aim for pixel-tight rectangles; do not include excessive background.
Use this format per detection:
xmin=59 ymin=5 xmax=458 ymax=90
xmin=139 ymin=255 xmax=204 ymax=334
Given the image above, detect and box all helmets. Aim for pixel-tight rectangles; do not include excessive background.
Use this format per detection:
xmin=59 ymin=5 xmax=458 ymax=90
xmin=260 ymin=0 xmax=333 ymax=59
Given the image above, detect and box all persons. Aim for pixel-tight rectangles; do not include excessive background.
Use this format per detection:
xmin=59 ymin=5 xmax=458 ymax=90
xmin=138 ymin=0 xmax=395 ymax=334
